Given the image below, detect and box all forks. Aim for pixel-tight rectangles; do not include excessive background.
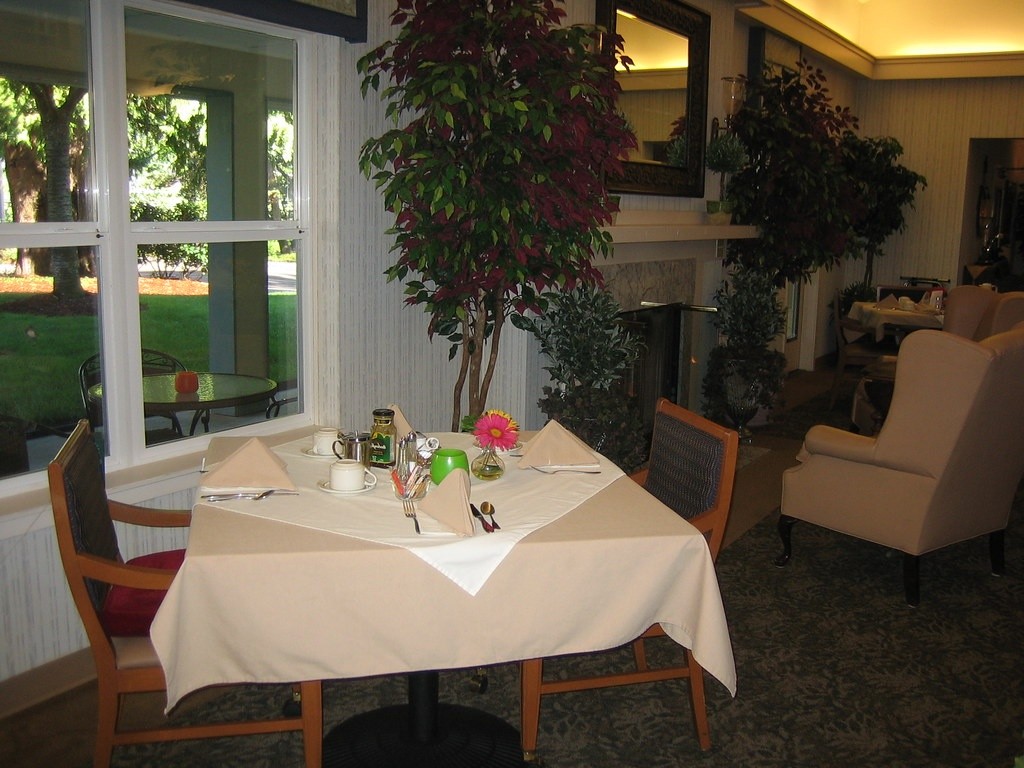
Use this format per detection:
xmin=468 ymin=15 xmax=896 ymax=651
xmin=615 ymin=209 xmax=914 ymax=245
xmin=402 ymin=498 xmax=421 ymax=534
xmin=528 ymin=464 xmax=601 ymax=476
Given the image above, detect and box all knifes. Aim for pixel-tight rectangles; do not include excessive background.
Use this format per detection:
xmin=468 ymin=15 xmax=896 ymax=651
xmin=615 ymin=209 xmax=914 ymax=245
xmin=469 ymin=503 xmax=493 ymax=534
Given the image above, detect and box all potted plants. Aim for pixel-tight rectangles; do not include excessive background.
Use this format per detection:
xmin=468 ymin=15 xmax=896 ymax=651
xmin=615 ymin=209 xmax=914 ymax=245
xmin=703 ymin=264 xmax=788 ymax=428
xmin=838 ymin=135 xmax=927 ymax=342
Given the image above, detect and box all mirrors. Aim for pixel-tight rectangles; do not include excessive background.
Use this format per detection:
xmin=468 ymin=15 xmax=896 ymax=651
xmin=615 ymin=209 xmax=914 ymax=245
xmin=595 ymin=0 xmax=713 ymax=201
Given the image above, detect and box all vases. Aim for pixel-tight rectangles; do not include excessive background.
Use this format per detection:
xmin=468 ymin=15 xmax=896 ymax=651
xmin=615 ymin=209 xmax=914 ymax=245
xmin=470 ymin=447 xmax=506 ymax=481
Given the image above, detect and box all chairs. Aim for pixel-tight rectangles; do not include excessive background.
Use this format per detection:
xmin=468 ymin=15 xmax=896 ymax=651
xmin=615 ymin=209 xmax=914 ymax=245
xmin=773 ymin=286 xmax=1024 ymax=610
xmin=520 ymin=398 xmax=739 ymax=751
xmin=78 ymin=349 xmax=187 ymax=445
xmin=47 ymin=420 xmax=325 ymax=767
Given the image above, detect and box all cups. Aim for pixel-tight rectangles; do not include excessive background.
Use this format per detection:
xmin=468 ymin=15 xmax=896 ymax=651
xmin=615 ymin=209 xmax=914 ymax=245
xmin=390 ymin=475 xmax=429 ymax=500
xmin=416 ymin=435 xmax=440 ymax=453
xmin=312 ymin=428 xmax=343 ymax=454
xmin=332 ymin=431 xmax=372 ymax=470
xmin=328 ymin=459 xmax=376 ymax=492
xmin=431 ymin=449 xmax=470 ymax=488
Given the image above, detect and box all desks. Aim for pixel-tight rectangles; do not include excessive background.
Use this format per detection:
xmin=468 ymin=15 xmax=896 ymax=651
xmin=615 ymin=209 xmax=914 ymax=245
xmin=151 ymin=426 xmax=737 ymax=768
xmin=87 ymin=373 xmax=277 ymax=441
xmin=844 ymin=302 xmax=943 ymax=353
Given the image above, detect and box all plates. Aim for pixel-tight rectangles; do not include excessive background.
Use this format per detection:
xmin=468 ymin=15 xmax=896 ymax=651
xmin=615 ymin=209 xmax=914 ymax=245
xmin=315 ymin=478 xmax=376 ymax=494
xmin=302 ymin=446 xmax=344 ymax=458
xmin=472 ymin=442 xmax=523 ymax=451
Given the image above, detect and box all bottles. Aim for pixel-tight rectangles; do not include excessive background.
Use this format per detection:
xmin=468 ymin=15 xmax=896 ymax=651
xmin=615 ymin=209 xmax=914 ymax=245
xmin=397 ymin=431 xmax=418 ymax=478
xmin=369 ymin=409 xmax=397 ymax=468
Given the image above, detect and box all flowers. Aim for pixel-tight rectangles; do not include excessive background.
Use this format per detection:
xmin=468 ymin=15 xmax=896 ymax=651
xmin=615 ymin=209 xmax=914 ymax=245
xmin=474 ymin=410 xmax=519 ymax=450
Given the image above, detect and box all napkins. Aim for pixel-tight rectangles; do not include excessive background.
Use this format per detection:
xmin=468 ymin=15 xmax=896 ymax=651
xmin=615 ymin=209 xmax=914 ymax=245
xmin=420 ymin=467 xmax=475 ymax=537
xmin=517 ymin=420 xmax=601 ymax=469
xmin=202 ymin=436 xmax=297 ymax=491
xmin=370 ymin=405 xmax=416 ymax=443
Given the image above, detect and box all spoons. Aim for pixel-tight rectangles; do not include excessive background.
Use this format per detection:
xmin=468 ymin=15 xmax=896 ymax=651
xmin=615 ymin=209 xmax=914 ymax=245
xmin=480 ymin=501 xmax=502 ymax=530
xmin=207 ymin=490 xmax=274 ymax=503
